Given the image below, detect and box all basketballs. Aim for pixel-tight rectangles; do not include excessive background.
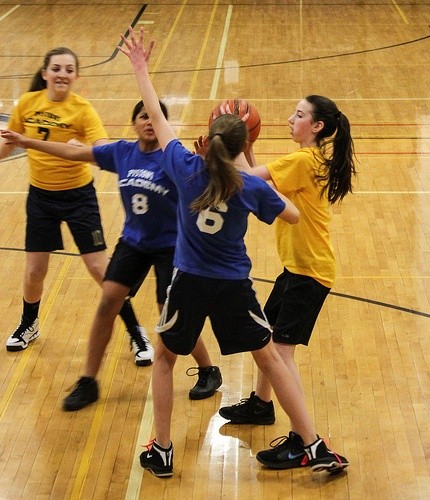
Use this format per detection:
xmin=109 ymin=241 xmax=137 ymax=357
xmin=208 ymin=99 xmax=262 ymax=144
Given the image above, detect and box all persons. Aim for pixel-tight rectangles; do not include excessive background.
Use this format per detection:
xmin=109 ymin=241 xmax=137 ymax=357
xmin=0 ymin=98 xmax=222 ymax=411
xmin=193 ymin=95 xmax=356 ymax=472
xmin=116 ymin=25 xmax=349 ymax=478
xmin=0 ymin=48 xmax=154 ymax=366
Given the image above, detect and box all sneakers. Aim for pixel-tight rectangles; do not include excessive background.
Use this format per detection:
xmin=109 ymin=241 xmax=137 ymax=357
xmin=63 ymin=377 xmax=99 ymax=411
xmin=303 ymin=438 xmax=349 ymax=473
xmin=140 ymin=438 xmax=173 ymax=477
xmin=126 ymin=326 xmax=155 ymax=366
xmin=256 ymin=431 xmax=320 ymax=467
xmin=6 ymin=314 xmax=41 ymax=352
xmin=219 ymin=391 xmax=276 ymax=425
xmin=186 ymin=366 xmax=222 ymax=400
